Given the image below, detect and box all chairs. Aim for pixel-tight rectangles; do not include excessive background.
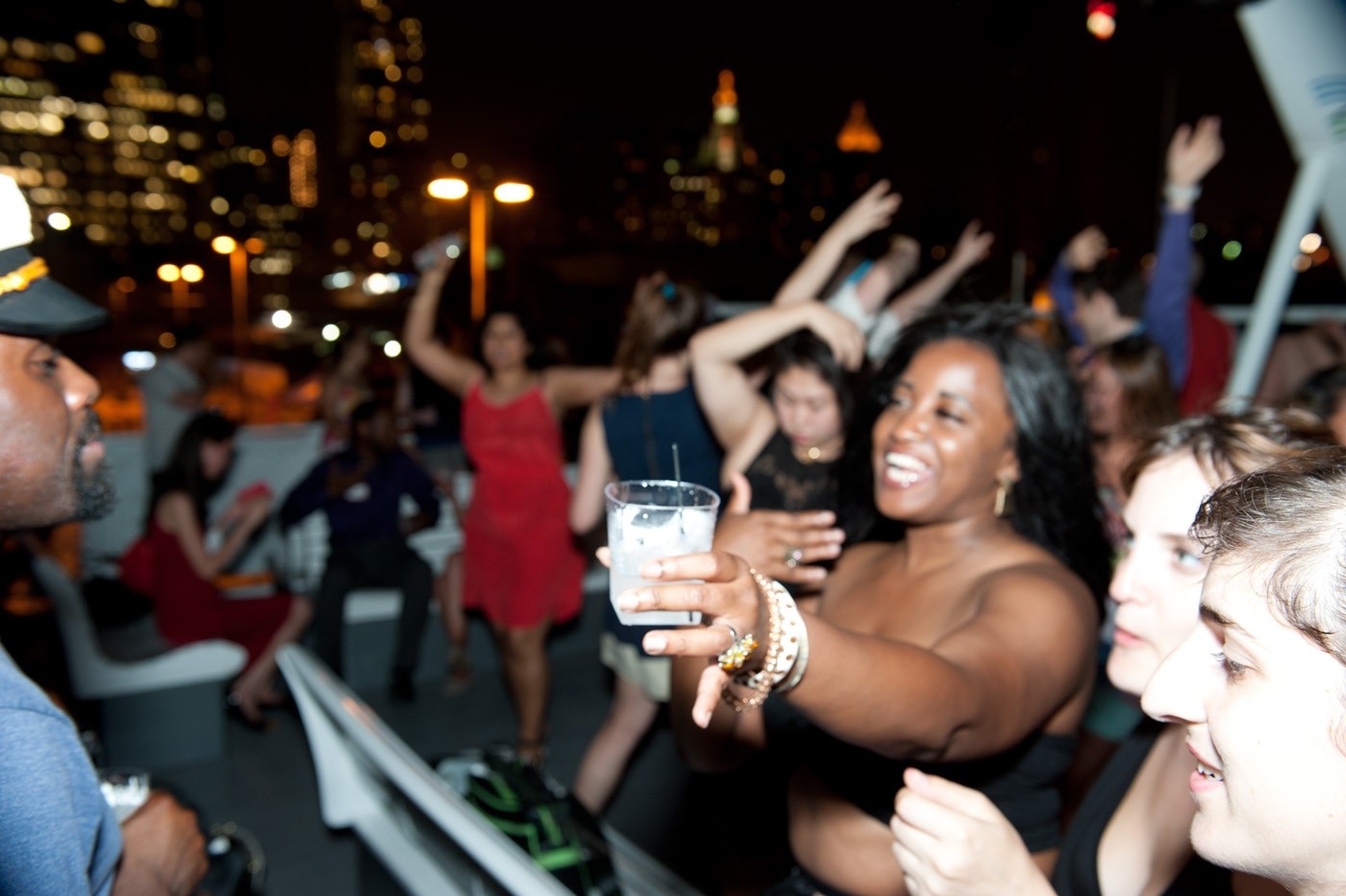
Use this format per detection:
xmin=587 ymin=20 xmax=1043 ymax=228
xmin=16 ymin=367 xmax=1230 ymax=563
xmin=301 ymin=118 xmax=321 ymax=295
xmin=37 ymin=559 xmax=249 ymax=778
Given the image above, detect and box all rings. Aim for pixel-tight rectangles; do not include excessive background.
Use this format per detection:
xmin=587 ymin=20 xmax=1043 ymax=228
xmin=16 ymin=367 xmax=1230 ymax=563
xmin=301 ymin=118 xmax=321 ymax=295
xmin=785 ymin=549 xmax=803 ymax=566
xmin=715 ymin=622 xmax=759 ymax=674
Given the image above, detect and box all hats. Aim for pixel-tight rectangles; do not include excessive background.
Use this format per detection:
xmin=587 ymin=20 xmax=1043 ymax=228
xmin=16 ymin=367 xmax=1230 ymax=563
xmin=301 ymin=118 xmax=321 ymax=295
xmin=0 ymin=174 xmax=108 ymax=331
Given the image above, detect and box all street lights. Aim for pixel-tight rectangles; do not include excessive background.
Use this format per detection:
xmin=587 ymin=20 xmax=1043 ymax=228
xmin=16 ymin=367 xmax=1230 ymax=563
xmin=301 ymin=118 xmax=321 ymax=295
xmin=212 ymin=235 xmax=266 ymax=327
xmin=429 ymin=167 xmax=535 ymax=326
xmin=158 ymin=263 xmax=204 ymax=326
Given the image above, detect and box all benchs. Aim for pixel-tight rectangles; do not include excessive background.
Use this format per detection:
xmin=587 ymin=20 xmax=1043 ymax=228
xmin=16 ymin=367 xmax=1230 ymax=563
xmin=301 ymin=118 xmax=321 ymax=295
xmin=83 ymin=418 xmax=405 ymax=623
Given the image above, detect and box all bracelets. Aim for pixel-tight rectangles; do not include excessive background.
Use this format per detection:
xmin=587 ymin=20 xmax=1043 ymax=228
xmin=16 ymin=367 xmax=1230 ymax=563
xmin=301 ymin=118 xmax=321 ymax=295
xmin=722 ymin=565 xmax=810 ymax=716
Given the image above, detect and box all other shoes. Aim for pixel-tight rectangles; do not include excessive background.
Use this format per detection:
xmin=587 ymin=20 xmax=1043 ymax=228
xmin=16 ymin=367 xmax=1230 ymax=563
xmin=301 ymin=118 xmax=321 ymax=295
xmin=226 ymin=692 xmax=266 ymax=734
xmin=390 ymin=679 xmax=418 ymax=700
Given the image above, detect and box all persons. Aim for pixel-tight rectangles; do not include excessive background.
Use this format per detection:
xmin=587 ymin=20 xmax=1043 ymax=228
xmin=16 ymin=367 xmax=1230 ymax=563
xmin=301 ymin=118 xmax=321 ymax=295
xmin=686 ymin=300 xmax=899 ymax=551
xmin=0 ymin=171 xmax=206 ymax=896
xmin=841 ymin=115 xmax=1224 ymax=369
xmin=400 ymin=238 xmax=622 ymax=766
xmin=887 ymin=357 xmax=1346 ymax=896
xmin=144 ymin=318 xmax=442 ymax=731
xmin=573 ymin=177 xmax=901 ymax=813
xmin=592 ymin=313 xmax=1115 ymax=895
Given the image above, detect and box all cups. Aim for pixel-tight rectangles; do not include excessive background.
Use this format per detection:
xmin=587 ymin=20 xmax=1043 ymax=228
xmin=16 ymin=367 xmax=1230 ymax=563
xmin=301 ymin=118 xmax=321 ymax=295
xmin=96 ymin=764 xmax=153 ymax=822
xmin=604 ymin=479 xmax=722 ymax=626
